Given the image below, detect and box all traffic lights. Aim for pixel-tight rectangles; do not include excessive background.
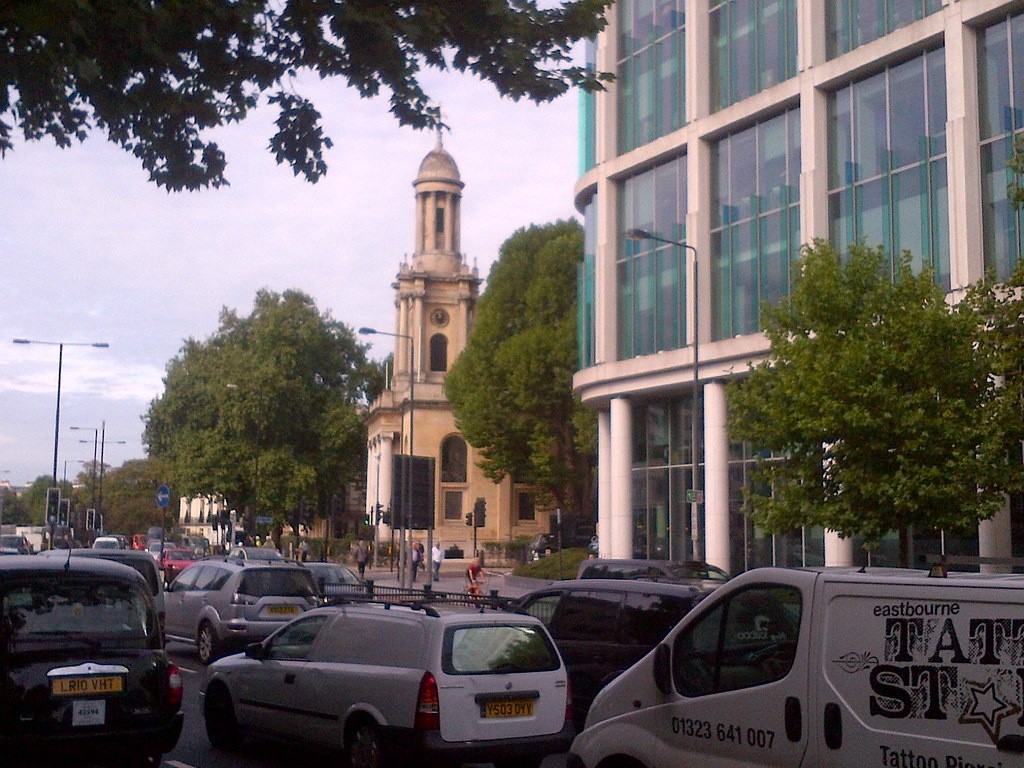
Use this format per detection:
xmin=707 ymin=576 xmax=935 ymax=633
xmin=46 ymin=486 xmax=61 ymax=525
xmin=376 ymin=503 xmax=383 ymax=523
xmin=466 ymin=512 xmax=472 ymax=526
xmin=60 ymin=500 xmax=70 ymax=528
xmin=86 ymin=509 xmax=95 ymax=531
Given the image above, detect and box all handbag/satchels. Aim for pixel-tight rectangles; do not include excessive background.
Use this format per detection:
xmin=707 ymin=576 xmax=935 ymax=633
xmin=418 ymin=559 xmax=425 ymax=569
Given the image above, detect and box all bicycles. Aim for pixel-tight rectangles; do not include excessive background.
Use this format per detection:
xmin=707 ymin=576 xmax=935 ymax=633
xmin=463 ymin=582 xmax=485 ymax=601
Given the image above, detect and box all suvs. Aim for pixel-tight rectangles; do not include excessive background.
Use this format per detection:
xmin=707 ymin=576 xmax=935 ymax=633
xmin=162 ymin=554 xmax=322 ymax=662
xmin=527 ymin=533 xmax=556 ymax=563
xmin=509 ymin=560 xmax=793 ymax=720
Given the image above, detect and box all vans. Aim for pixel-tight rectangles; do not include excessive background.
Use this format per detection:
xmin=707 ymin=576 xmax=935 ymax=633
xmin=576 ymin=559 xmax=734 ymax=583
xmin=38 ymin=548 xmax=165 ymax=637
xmin=197 ymin=594 xmax=569 ymax=768
xmin=565 ymin=565 xmax=1024 ymax=766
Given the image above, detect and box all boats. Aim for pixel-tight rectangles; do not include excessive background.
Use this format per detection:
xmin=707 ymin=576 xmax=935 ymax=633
xmin=364 ymin=514 xmax=369 ymax=525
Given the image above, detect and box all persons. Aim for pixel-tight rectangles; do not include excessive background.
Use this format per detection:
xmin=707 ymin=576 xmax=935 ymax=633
xmin=60 ymin=534 xmax=76 ymax=549
xmin=587 ymin=553 xmax=596 ymax=562
xmin=352 ymin=539 xmax=372 ymax=579
xmin=299 ymin=539 xmax=310 ymax=563
xmin=410 ymin=540 xmax=425 ymax=583
xmin=243 ymin=533 xmax=276 ymax=550
xmin=431 ymin=541 xmax=443 ymax=582
xmin=464 ymin=558 xmax=483 ymax=605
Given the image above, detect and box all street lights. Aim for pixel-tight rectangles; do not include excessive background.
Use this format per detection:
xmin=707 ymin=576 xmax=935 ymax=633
xmin=627 ymin=229 xmax=699 ymax=569
xmin=70 ymin=426 xmax=98 ymax=544
xmin=62 ymin=460 xmax=84 ymax=539
xmin=80 ymin=439 xmax=125 ymax=545
xmin=359 ymin=328 xmax=414 ymax=588
xmin=13 ymin=338 xmax=111 ymax=545
xmin=397 ymin=397 xmax=410 ymax=586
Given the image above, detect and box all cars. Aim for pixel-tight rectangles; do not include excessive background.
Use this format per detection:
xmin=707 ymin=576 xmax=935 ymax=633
xmin=0 ymin=535 xmax=33 ymax=554
xmin=305 ymin=561 xmax=376 ymax=605
xmin=226 ymin=546 xmax=287 ymax=563
xmin=92 ymin=534 xmax=212 ymax=580
xmin=1 ymin=553 xmax=184 ymax=767
